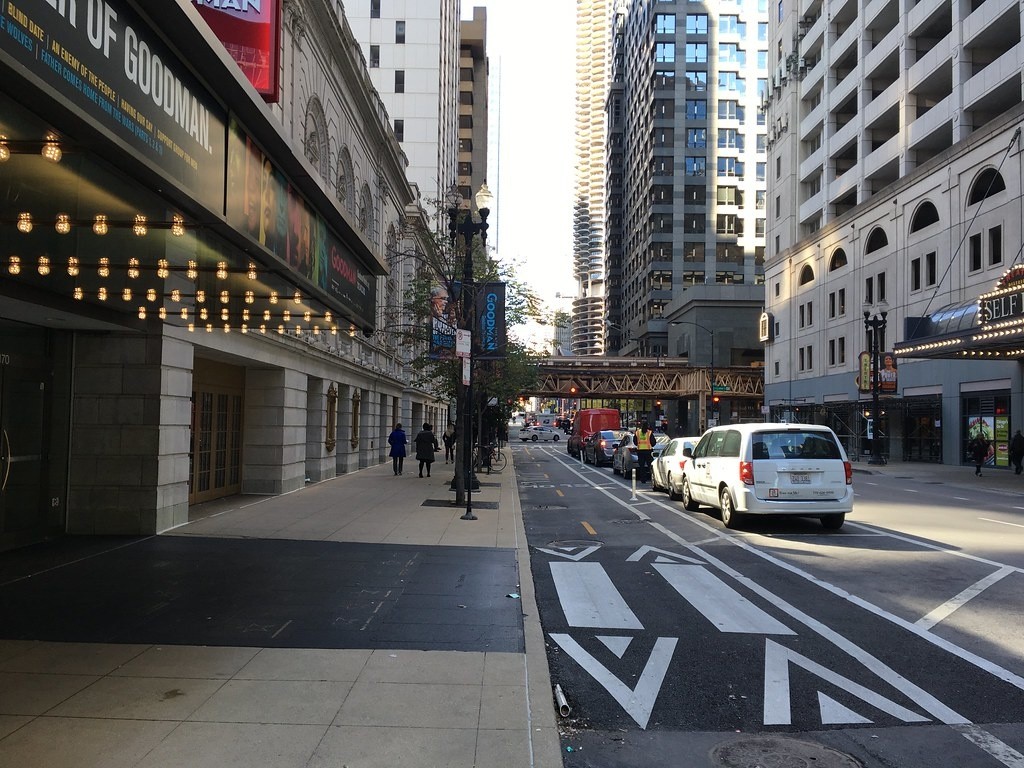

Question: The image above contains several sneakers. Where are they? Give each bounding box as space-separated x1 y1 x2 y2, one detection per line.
978 472 982 477
973 472 978 477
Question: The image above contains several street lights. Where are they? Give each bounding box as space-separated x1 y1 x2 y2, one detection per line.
445 173 496 491
863 295 890 467
671 321 715 419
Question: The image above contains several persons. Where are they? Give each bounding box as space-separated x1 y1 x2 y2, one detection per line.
388 422 407 476
1008 428 1024 475
228 115 329 292
879 354 897 391
634 421 656 483
413 423 436 478
442 423 456 464
969 433 990 478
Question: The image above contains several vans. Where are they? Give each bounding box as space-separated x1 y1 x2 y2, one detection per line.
565 407 620 457
683 423 854 530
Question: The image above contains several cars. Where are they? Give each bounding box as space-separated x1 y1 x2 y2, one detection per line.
585 430 631 468
649 437 702 500
518 426 561 442
612 433 671 479
520 408 575 435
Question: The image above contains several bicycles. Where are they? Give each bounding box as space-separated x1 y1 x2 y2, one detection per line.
472 442 507 472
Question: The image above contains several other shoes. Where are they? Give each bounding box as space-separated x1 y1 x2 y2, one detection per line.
398 473 403 475
427 474 431 477
393 473 398 476
419 474 424 478
446 462 449 464
451 461 454 464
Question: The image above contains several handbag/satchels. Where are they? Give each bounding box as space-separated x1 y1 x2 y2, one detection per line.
451 445 456 450
